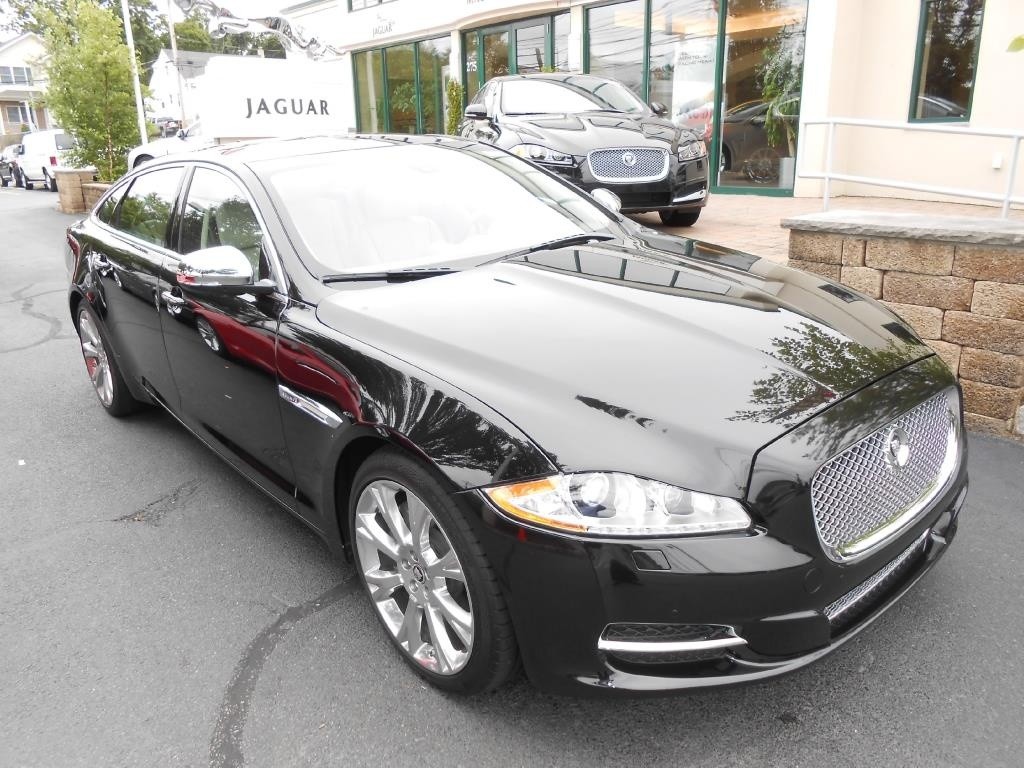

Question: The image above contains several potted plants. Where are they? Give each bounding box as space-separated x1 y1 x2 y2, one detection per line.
754 35 805 189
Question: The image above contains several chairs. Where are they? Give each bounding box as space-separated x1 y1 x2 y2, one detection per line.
200 194 263 281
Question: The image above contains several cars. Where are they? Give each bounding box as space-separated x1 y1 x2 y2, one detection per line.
460 72 710 226
724 91 970 186
66 116 973 696
0 144 25 188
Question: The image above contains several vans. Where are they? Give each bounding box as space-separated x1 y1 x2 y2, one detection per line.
13 129 99 192
